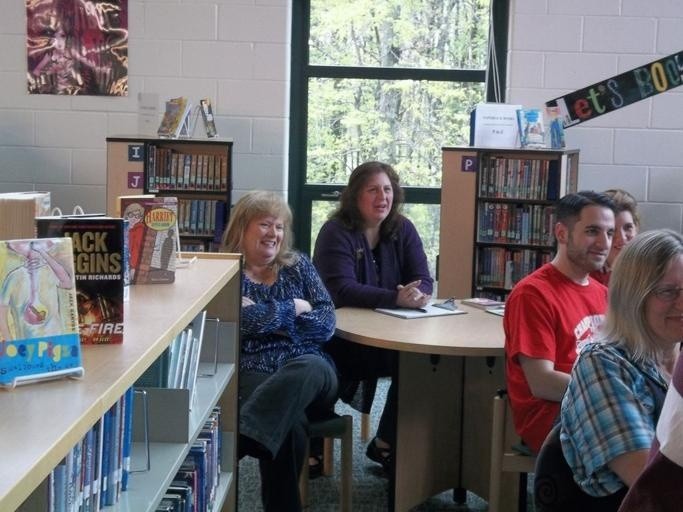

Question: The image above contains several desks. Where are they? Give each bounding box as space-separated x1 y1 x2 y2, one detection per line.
332 299 523 512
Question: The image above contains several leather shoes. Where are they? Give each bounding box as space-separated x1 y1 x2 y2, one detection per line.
309 456 324 480
367 437 396 466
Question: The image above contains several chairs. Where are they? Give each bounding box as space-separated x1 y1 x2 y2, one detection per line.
484 384 546 512
528 419 630 512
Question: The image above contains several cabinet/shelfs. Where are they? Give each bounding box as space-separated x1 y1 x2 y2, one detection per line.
103 133 236 254
435 144 580 306
0 250 243 512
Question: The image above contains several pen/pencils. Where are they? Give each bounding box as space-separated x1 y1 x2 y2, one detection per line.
417 308 426 312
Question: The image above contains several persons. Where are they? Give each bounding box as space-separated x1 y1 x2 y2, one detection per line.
28 15 96 94
503 191 619 460
615 349 682 512
220 192 338 512
311 161 438 481
591 187 641 288
534 228 681 512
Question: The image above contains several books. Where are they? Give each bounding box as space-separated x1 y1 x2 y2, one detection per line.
148 94 231 252
486 304 505 317
117 194 181 284
374 302 469 320
15 310 234 512
0 213 129 385
473 98 566 299
461 296 499 310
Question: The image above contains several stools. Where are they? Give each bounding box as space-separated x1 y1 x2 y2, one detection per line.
345 365 392 444
295 402 360 512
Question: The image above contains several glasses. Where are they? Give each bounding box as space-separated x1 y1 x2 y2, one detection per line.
650 285 683 303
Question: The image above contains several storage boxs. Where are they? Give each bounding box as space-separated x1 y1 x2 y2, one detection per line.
467 101 522 149
